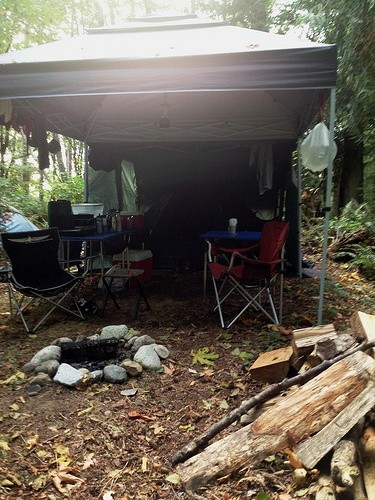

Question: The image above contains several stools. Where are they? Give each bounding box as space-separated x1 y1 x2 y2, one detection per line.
103 268 151 319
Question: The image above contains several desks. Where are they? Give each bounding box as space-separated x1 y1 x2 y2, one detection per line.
200 231 276 304
59 231 129 290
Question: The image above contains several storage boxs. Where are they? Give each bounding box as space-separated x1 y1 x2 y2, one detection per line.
120 211 145 229
113 250 152 287
72 203 104 218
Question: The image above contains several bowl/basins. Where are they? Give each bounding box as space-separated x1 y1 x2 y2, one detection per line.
75 226 96 236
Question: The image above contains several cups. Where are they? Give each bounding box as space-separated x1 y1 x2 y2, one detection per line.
97 215 106 233
228 219 237 234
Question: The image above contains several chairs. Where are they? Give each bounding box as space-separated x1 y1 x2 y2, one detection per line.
0 226 86 333
204 219 291 330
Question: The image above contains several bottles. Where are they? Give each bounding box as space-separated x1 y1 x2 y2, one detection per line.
113 210 121 232
